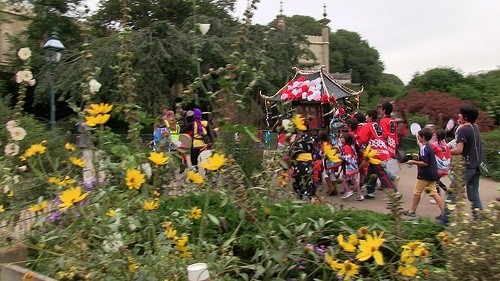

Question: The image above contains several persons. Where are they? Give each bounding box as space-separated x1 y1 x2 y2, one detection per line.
401 127 444 218
179 102 483 225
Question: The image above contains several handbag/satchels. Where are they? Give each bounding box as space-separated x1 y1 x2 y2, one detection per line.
385 158 401 173
479 161 490 176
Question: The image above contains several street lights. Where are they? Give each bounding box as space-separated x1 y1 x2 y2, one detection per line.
42 38 66 130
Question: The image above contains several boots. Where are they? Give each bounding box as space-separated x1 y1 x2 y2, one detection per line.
278 176 288 187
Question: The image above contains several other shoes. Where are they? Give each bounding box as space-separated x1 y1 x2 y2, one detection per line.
402 210 416 217
341 191 353 198
393 176 400 183
436 215 445 220
430 199 437 204
354 194 364 200
363 195 375 199
326 188 338 196
